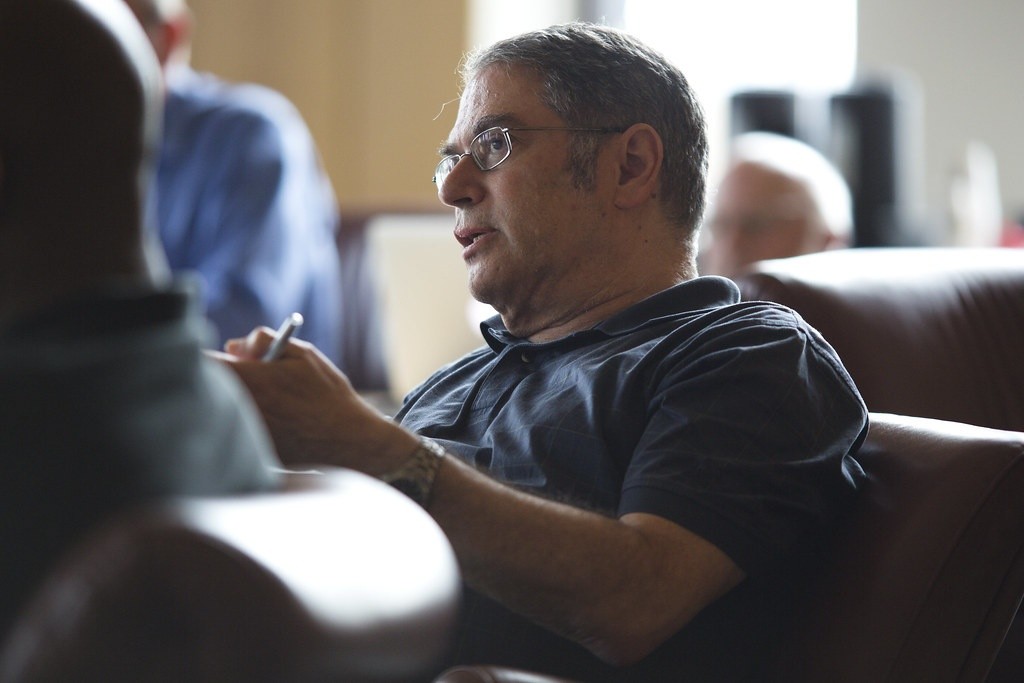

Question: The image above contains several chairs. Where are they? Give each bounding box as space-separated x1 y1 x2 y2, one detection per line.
3 213 1024 682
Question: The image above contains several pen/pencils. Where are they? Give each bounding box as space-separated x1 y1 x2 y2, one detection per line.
261 312 303 363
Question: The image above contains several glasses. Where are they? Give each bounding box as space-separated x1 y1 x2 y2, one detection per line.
433 126 633 196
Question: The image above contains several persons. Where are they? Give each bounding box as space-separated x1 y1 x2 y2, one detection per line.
0 0 277 612
697 130 854 282
130 0 339 361
204 21 870 683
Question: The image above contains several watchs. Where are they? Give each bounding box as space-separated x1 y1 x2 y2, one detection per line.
378 433 444 510
